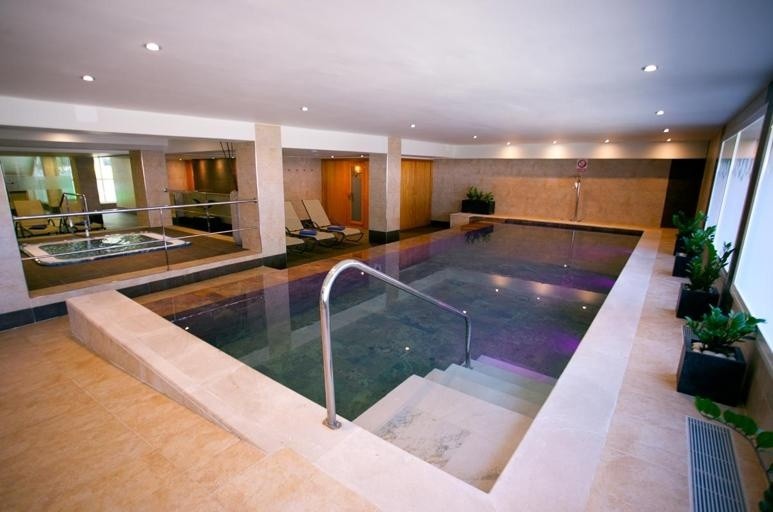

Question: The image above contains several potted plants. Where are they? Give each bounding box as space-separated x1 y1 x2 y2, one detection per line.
677 243 736 322
671 211 718 279
676 308 766 406
460 186 496 215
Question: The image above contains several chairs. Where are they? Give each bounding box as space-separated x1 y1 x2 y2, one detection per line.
11 195 104 238
284 197 365 253
